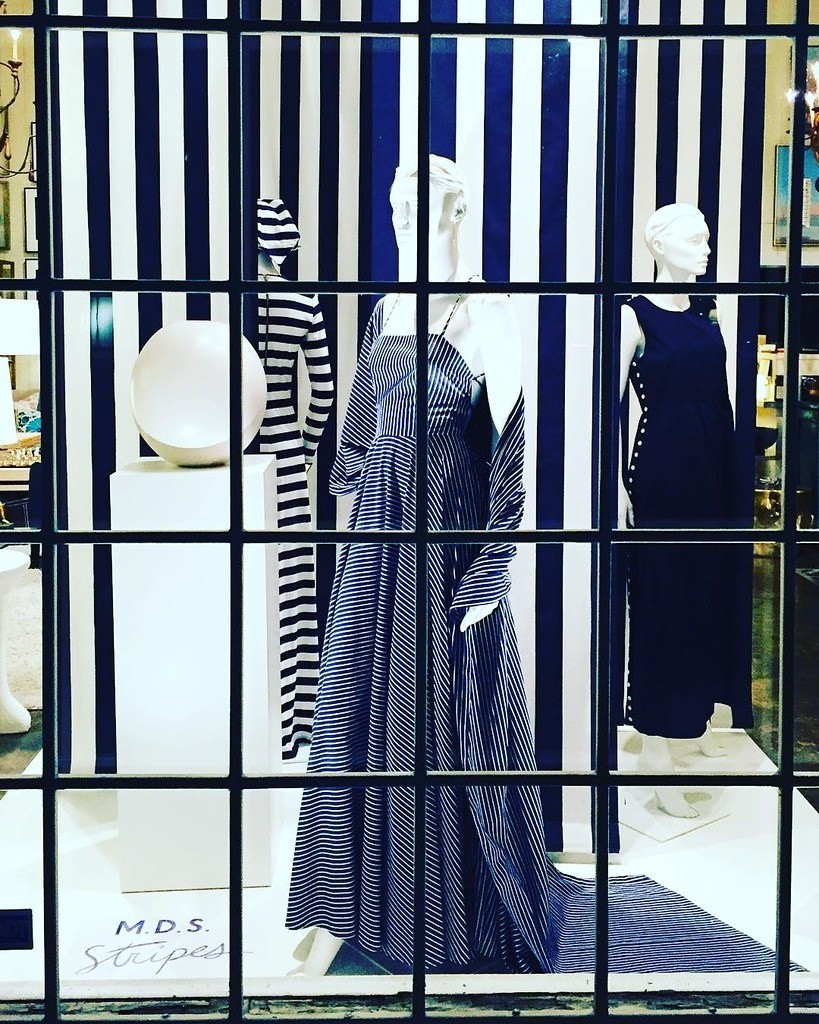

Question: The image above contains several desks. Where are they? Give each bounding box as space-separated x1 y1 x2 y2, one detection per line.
0 550 31 735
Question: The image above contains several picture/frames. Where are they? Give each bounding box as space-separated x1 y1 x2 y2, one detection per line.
24 187 40 253
30 121 37 183
790 45 819 84
772 143 819 251
24 258 39 299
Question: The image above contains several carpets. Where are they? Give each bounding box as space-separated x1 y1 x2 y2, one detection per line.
3 546 44 709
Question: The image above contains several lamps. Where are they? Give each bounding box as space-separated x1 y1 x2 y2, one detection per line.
786 106 819 195
0 30 37 180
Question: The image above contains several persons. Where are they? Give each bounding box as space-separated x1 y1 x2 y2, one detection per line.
9 361 39 429
614 201 758 818
253 197 333 761
284 152 809 999
